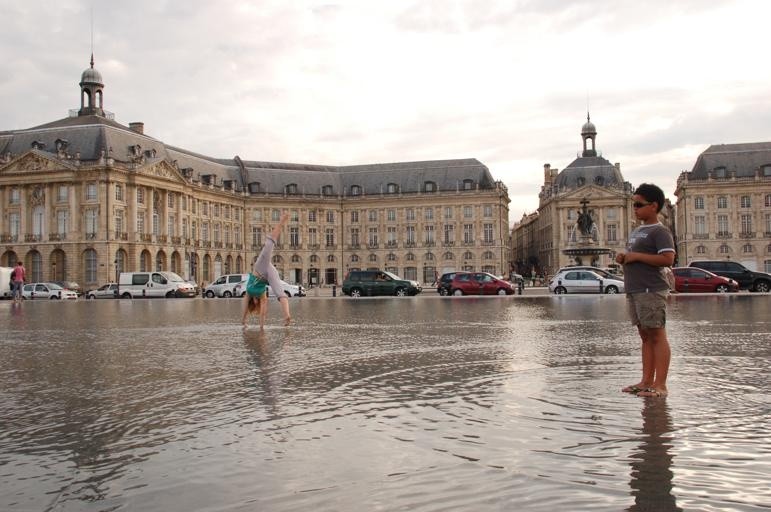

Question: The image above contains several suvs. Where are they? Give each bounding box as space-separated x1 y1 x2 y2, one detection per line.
555 265 624 281
688 259 771 293
342 270 422 297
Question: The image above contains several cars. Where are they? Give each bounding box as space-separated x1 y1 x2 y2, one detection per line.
21 282 78 300
669 267 741 293
88 282 119 298
436 271 468 296
548 269 626 294
452 272 516 296
46 280 82 295
236 275 307 297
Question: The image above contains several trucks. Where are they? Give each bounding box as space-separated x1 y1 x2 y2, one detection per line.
1 266 25 300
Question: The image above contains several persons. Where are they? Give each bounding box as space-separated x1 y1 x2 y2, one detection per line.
241 211 291 330
9 266 20 300
12 262 26 304
615 184 676 397
531 270 548 287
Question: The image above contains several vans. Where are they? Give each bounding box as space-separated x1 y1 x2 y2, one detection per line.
118 270 196 297
203 274 250 297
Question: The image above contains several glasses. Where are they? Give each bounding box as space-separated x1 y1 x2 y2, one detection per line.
634 201 651 208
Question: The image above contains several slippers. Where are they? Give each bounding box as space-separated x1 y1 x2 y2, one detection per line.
622 385 669 398
